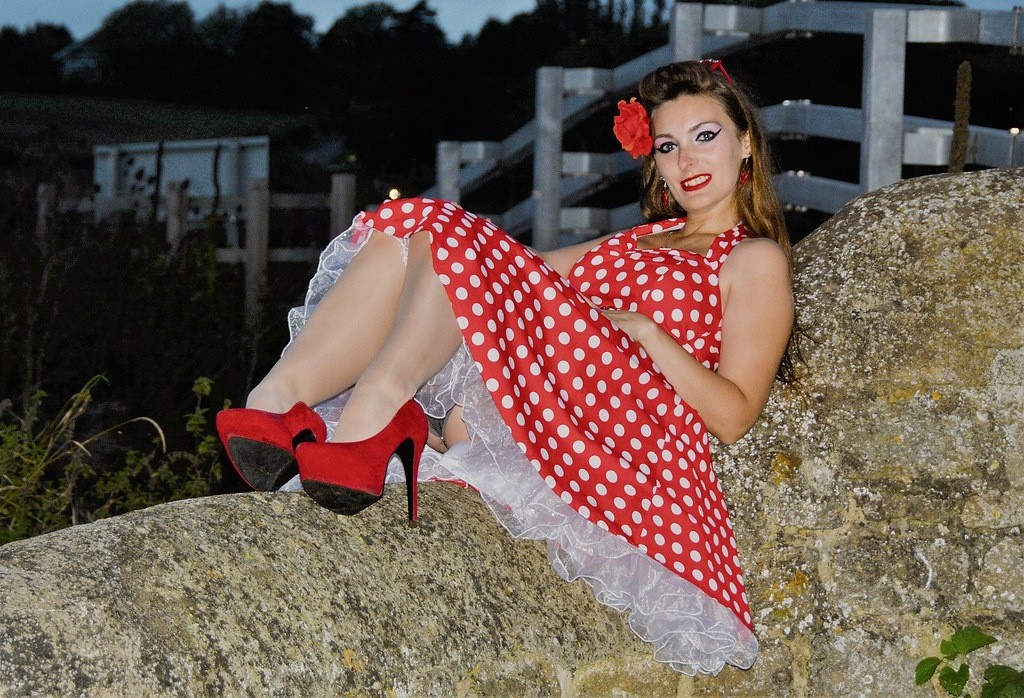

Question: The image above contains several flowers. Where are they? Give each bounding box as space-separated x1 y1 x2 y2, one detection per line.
612 97 654 159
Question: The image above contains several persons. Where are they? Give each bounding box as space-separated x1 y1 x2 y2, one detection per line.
217 61 797 678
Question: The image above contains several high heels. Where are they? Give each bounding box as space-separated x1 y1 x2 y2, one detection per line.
295 400 429 528
216 401 327 492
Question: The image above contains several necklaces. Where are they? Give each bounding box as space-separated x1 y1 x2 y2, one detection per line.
666 229 711 249
